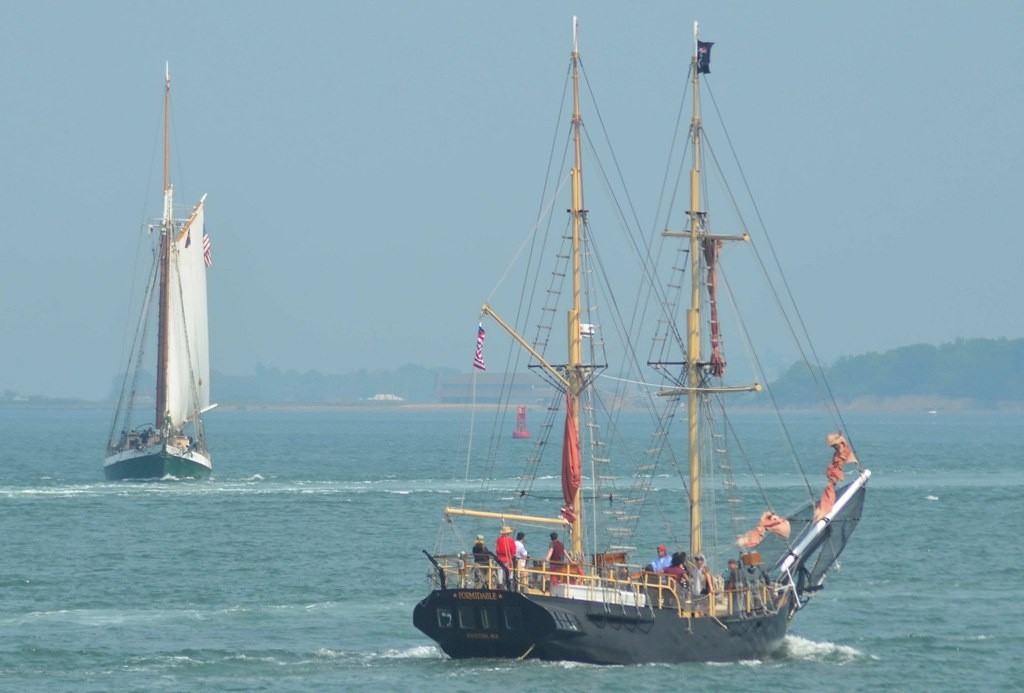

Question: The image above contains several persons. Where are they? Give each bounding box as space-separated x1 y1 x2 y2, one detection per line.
189 438 199 452
644 545 770 600
496 527 516 592
472 534 494 581
114 427 156 452
514 532 528 590
545 533 577 593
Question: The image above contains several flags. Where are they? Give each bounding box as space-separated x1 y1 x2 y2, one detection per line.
203 223 212 268
698 39 715 73
474 322 486 370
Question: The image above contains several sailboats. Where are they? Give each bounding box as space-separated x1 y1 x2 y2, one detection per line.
414 13 872 667
102 57 217 483
514 406 530 437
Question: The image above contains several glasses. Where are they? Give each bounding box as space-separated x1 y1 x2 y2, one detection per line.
658 550 661 552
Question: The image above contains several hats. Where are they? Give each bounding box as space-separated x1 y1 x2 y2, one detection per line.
672 552 685 564
728 560 734 564
695 554 703 560
500 526 512 534
516 532 525 539
549 533 557 538
657 545 665 551
474 534 484 544
